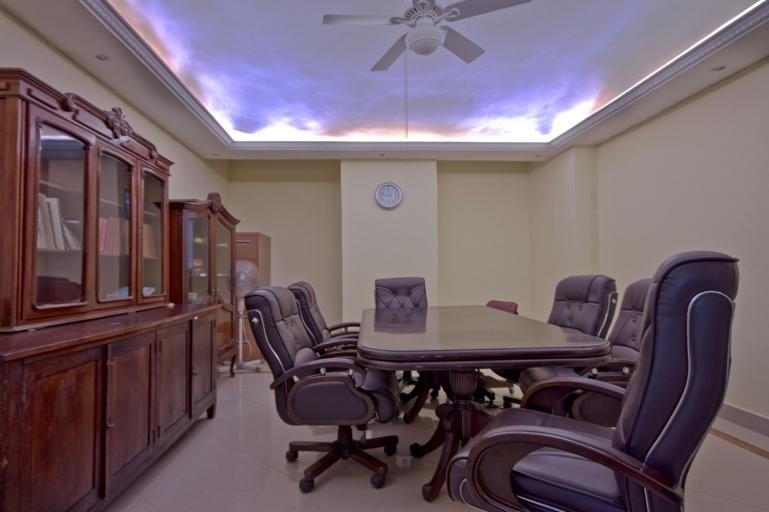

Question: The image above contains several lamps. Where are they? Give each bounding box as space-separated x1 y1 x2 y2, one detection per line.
404 17 445 57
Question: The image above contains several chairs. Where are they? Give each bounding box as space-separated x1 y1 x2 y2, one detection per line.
485 299 519 392
288 281 395 430
374 276 440 397
519 279 655 428
490 274 618 409
444 249 740 512
244 285 399 494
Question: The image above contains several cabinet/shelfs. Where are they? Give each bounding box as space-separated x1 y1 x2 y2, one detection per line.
168 189 240 380
235 229 270 364
0 68 177 334
1 301 225 512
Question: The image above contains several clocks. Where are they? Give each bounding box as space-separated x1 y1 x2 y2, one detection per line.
375 181 402 210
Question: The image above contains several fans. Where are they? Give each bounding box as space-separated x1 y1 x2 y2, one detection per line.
322 0 531 72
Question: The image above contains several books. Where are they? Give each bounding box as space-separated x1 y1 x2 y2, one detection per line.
32 183 160 262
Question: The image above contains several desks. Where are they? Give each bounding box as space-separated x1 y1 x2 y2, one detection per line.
357 305 613 502
